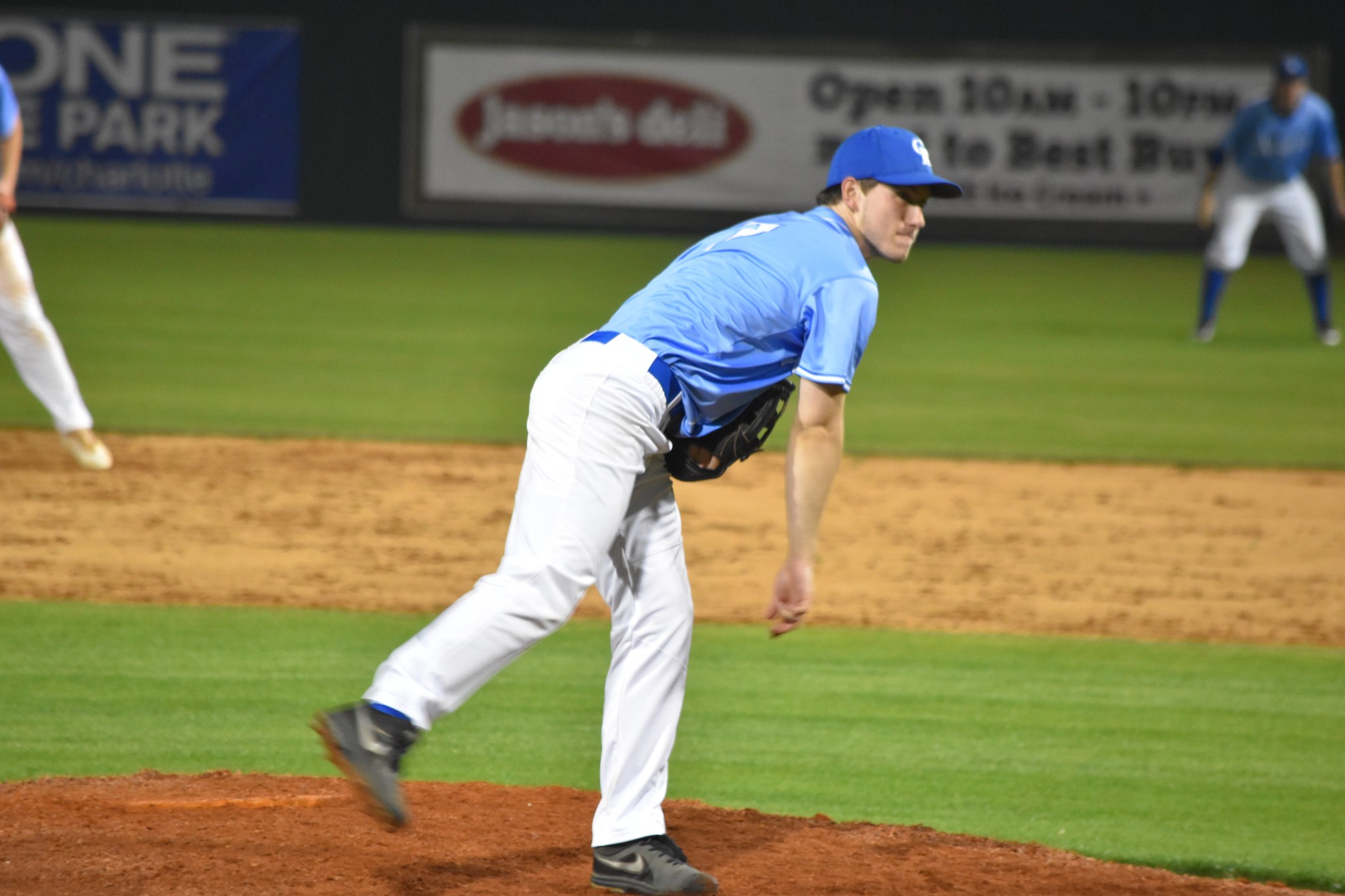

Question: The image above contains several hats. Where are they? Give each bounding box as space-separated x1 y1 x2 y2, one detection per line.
1278 58 1306 82
826 124 964 198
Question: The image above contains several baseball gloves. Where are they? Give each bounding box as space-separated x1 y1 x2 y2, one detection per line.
664 375 796 481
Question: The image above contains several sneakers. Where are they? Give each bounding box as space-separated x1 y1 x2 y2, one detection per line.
590 834 719 896
313 698 412 830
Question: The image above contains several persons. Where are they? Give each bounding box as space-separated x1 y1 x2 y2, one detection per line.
311 124 962 896
1193 55 1344 344
0 68 111 470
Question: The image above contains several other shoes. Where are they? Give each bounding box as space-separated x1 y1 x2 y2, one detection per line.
1318 326 1340 345
66 427 114 471
1194 323 1214 342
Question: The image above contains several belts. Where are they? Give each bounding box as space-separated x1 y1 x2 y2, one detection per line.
584 329 685 439
